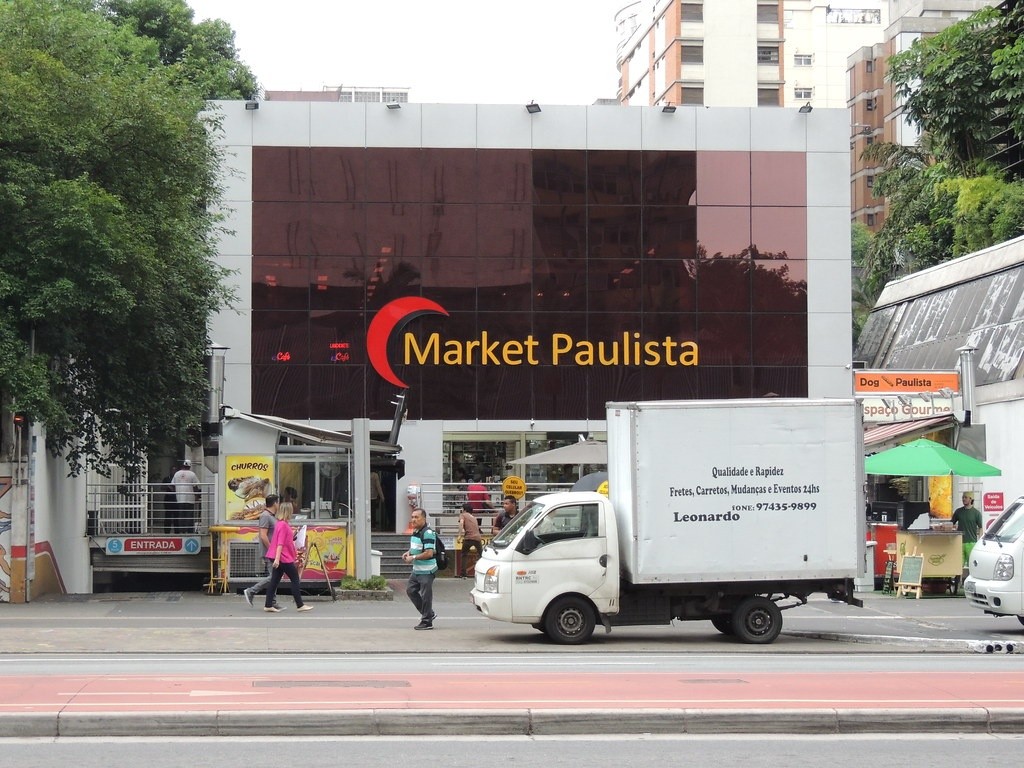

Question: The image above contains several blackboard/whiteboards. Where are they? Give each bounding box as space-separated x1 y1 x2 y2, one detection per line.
898 555 924 587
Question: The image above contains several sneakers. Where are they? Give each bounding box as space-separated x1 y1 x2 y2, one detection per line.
432 613 437 620
460 575 467 579
274 605 287 611
244 589 253 607
297 605 314 613
414 621 434 630
264 607 279 612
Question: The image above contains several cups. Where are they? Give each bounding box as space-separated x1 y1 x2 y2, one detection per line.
882 511 887 523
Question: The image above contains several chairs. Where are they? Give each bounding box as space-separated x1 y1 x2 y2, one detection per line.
582 512 596 537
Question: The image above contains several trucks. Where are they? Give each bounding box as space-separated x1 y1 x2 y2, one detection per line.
470 397 867 645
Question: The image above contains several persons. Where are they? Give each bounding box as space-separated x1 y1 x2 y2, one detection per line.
282 487 301 513
172 460 200 533
244 494 281 612
263 502 314 612
161 466 180 534
467 474 497 514
946 490 983 584
454 467 469 491
473 456 493 476
402 508 438 630
457 504 484 580
369 466 385 531
492 495 522 534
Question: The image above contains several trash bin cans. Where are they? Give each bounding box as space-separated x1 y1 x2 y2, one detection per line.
852 540 878 593
371 549 383 576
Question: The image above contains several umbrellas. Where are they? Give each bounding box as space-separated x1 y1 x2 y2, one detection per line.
864 436 1001 501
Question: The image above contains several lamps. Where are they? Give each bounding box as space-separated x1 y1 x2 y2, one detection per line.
897 395 912 418
244 96 259 110
846 363 851 370
387 100 400 109
662 101 677 112
938 387 954 412
919 392 935 414
526 99 541 113
881 398 896 420
798 100 812 113
531 419 534 427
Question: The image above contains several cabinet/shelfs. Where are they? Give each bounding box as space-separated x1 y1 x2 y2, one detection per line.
441 442 517 508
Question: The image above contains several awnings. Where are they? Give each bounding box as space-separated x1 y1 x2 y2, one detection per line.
864 412 958 445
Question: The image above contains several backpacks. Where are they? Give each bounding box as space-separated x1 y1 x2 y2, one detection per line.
420 528 447 569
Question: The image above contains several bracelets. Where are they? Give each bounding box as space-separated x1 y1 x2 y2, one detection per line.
413 555 417 560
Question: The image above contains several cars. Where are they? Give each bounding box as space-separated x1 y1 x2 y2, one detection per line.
963 497 1024 626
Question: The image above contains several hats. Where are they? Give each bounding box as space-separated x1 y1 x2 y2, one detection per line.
963 491 974 499
183 460 192 466
